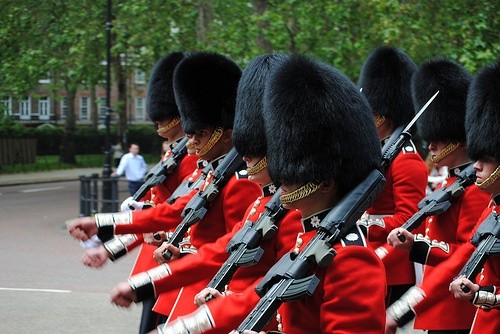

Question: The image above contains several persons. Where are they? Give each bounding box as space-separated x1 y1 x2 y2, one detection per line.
110 144 147 197
65 48 500 334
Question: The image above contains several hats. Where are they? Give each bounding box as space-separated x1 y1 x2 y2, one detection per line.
359 45 419 126
465 59 499 158
147 50 179 121
261 51 383 191
232 51 281 159
410 56 475 144
173 50 243 135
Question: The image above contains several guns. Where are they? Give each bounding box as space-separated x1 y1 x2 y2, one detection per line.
127 136 190 210
452 209 500 293
161 146 246 260
234 90 441 333
380 117 418 173
394 159 477 243
202 184 286 302
